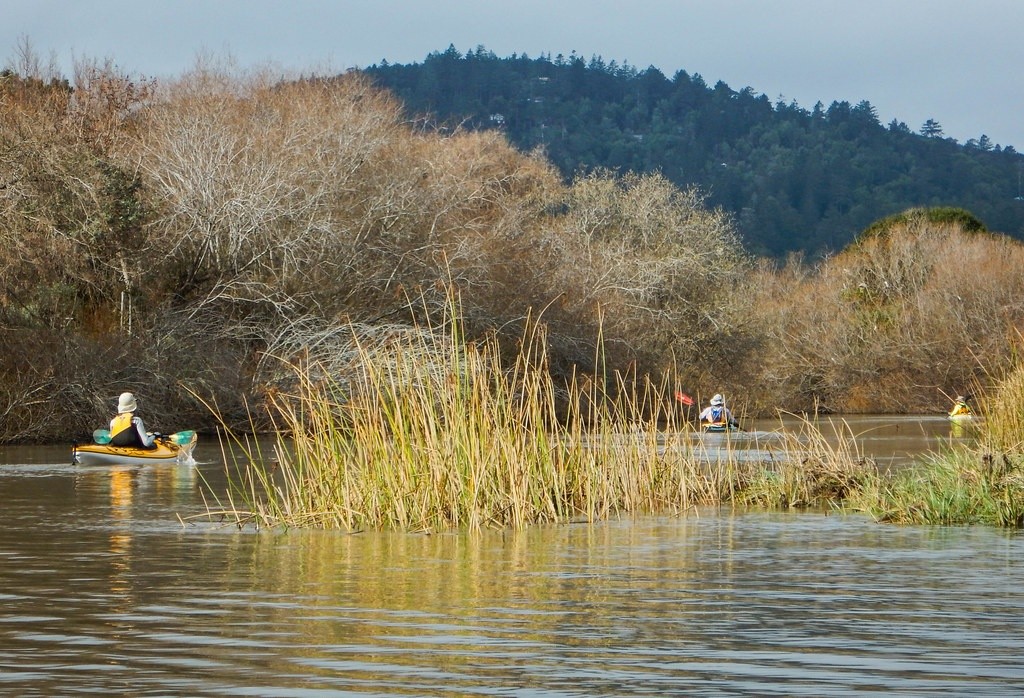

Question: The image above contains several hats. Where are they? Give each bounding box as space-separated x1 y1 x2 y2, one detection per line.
118 392 138 414
955 395 965 402
710 393 724 406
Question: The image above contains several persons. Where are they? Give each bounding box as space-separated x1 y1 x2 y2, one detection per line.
951 419 965 437
109 392 163 450
951 395 972 416
698 393 742 429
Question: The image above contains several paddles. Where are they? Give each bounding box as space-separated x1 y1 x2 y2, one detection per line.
92 428 197 446
674 390 748 433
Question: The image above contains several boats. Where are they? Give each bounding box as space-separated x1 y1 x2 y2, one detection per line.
703 421 732 432
71 430 199 467
948 412 972 422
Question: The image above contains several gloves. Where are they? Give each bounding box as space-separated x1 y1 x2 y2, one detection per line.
153 431 163 439
